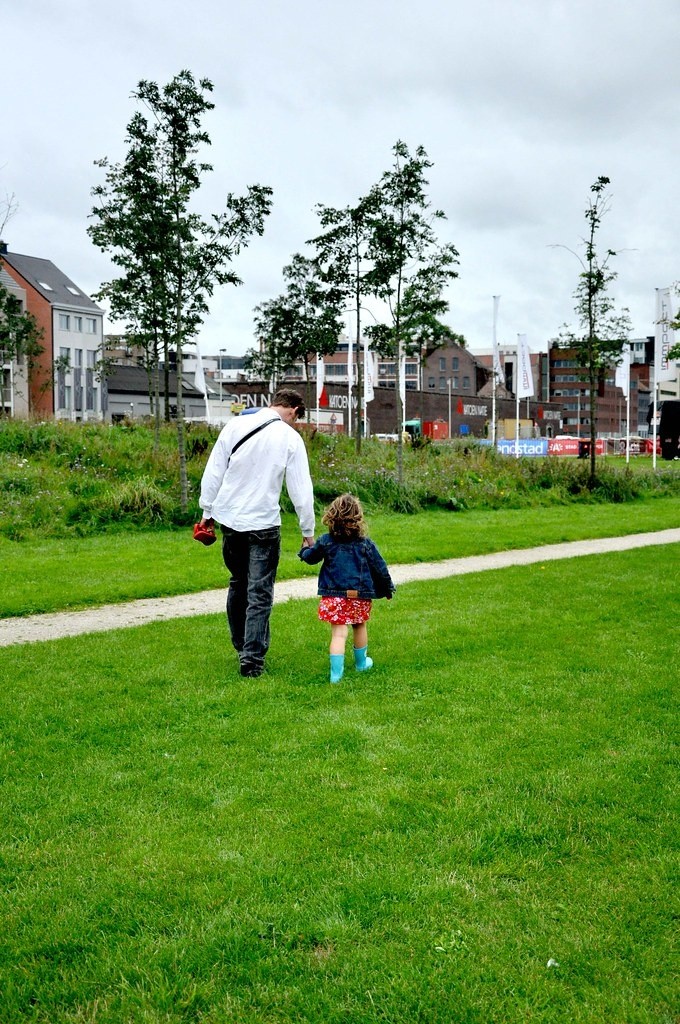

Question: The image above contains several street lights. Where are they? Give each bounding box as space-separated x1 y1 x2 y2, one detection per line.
219 349 226 416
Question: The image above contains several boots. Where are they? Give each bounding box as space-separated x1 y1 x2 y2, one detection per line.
330 653 344 683
353 644 373 670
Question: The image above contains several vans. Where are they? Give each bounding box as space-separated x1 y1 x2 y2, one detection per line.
398 419 421 440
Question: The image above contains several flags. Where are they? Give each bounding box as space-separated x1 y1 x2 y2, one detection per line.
654 288 674 383
194 351 207 395
398 342 406 404
364 340 374 402
516 341 535 399
316 354 328 409
492 341 505 390
614 342 628 399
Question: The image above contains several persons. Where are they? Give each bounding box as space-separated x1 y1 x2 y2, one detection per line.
196 387 315 678
296 494 395 686
